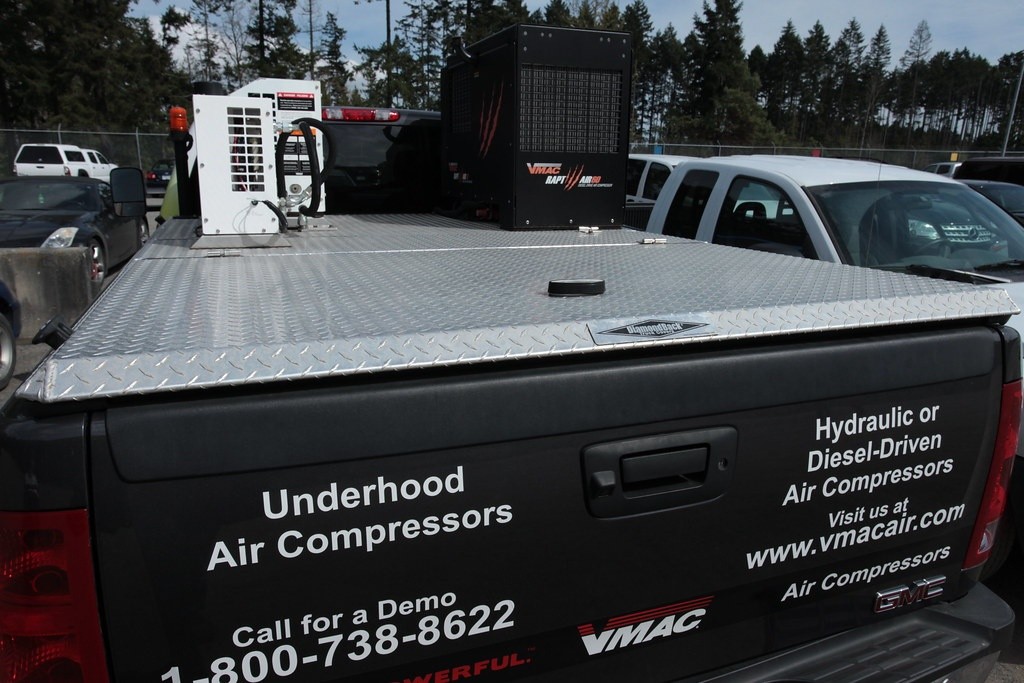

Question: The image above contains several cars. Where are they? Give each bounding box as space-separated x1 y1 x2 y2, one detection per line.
145 160 175 195
0 175 151 288
954 179 1024 229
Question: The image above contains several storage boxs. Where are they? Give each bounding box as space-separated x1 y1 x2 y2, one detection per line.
441 22 631 232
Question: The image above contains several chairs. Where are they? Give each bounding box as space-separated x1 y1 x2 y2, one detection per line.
732 199 767 227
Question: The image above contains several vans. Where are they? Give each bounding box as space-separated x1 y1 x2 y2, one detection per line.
13 143 119 185
626 154 704 203
922 157 1024 187
645 154 1024 458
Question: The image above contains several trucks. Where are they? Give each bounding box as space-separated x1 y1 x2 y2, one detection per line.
2 106 1023 683
